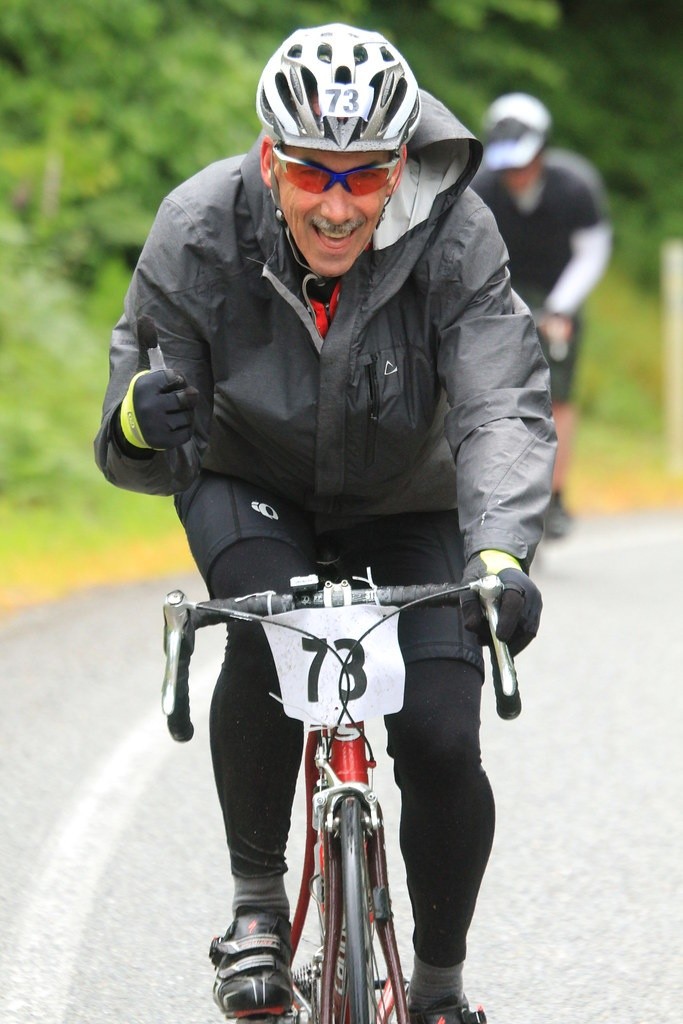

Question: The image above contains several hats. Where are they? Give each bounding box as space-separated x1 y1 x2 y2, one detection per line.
483 133 545 173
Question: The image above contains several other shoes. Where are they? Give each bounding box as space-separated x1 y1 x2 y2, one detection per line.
539 491 568 541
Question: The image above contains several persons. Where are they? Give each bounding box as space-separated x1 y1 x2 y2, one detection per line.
470 93 613 539
92 22 562 1024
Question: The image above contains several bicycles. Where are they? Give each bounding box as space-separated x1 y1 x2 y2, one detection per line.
159 572 525 1023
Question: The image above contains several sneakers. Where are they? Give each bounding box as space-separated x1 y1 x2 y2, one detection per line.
209 905 294 1018
404 985 487 1024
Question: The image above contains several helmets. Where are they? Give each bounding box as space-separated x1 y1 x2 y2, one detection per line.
256 24 420 152
478 93 552 139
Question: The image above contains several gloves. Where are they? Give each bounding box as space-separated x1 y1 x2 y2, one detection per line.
459 547 542 657
109 313 199 460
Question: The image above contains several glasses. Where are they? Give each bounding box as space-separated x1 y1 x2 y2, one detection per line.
273 140 403 195
